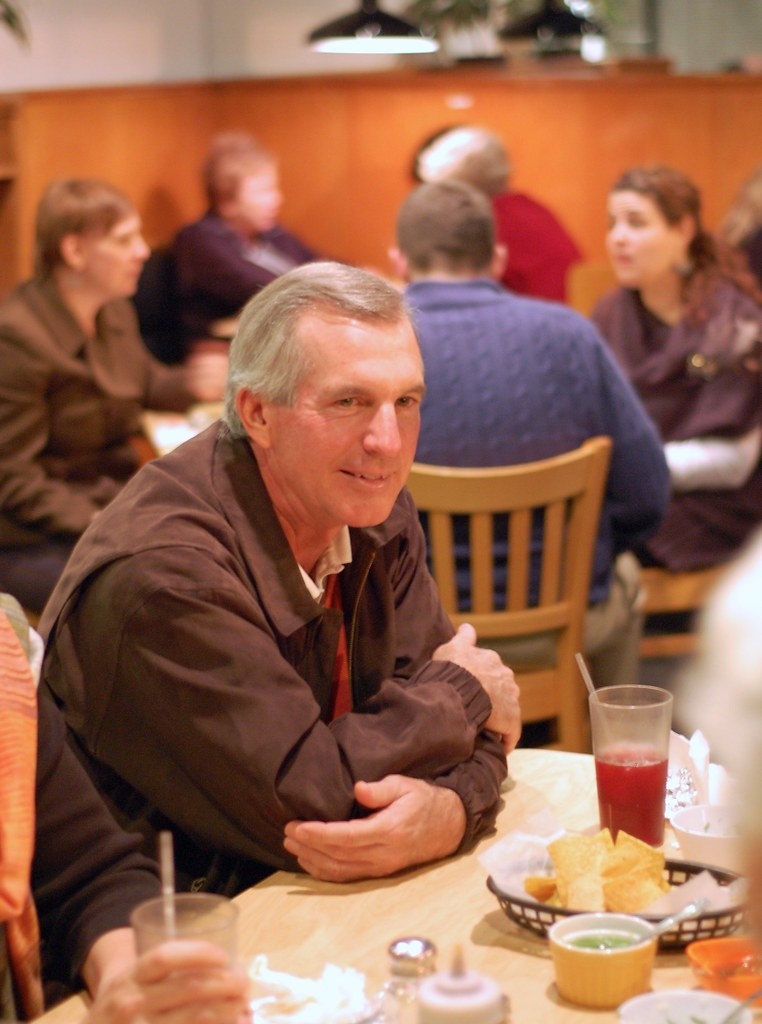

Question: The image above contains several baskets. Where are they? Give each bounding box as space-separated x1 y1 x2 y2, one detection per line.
487 858 747 952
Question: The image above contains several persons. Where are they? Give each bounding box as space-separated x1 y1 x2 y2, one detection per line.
0 178 229 617
36 260 521 899
0 593 251 1024
589 163 762 634
389 182 670 695
137 134 322 370
388 122 584 303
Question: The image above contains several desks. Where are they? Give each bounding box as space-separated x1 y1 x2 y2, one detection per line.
16 744 709 1024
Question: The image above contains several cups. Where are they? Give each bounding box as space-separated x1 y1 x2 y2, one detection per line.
589 684 675 848
618 989 753 1024
130 892 239 970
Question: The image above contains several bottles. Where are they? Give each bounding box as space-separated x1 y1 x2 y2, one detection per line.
382 936 438 1023
417 944 503 1023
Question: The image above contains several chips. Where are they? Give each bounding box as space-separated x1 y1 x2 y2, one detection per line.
521 827 674 913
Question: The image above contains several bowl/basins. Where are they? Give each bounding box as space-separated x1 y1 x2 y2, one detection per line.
670 803 743 875
547 913 658 1010
687 935 762 1008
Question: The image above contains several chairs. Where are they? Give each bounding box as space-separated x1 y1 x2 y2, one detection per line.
413 436 611 749
0 587 50 1024
640 565 732 679
